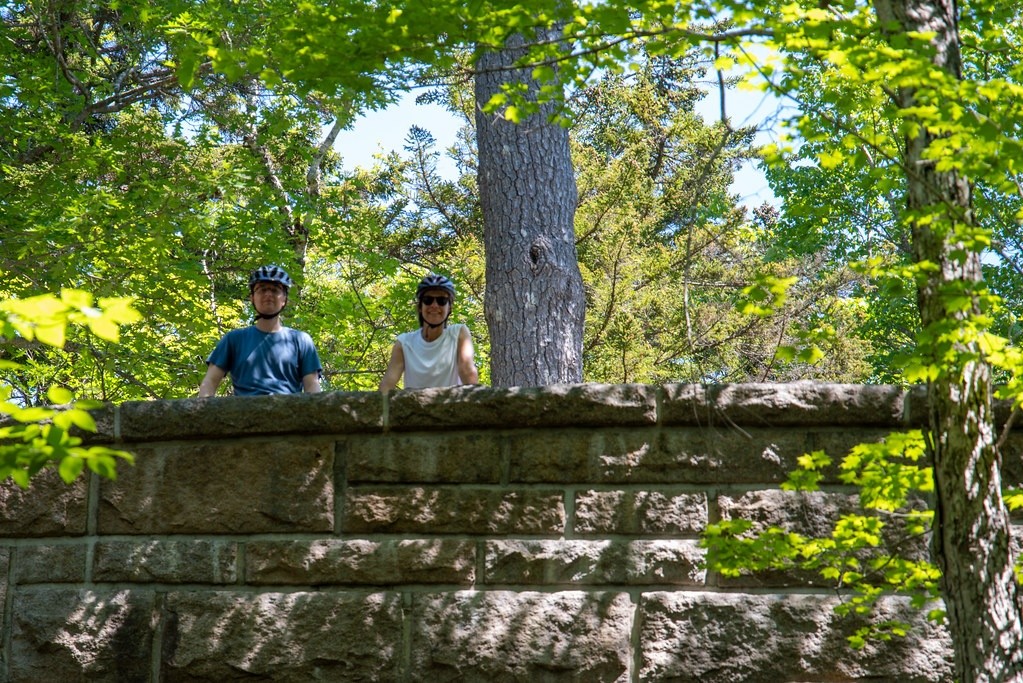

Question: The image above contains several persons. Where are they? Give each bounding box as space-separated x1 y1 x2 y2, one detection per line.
200 265 323 397
379 275 478 388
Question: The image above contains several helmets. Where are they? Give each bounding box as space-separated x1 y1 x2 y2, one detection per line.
417 276 455 296
249 264 296 287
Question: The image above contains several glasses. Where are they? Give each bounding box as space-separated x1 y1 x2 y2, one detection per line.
254 285 286 295
421 295 450 306
